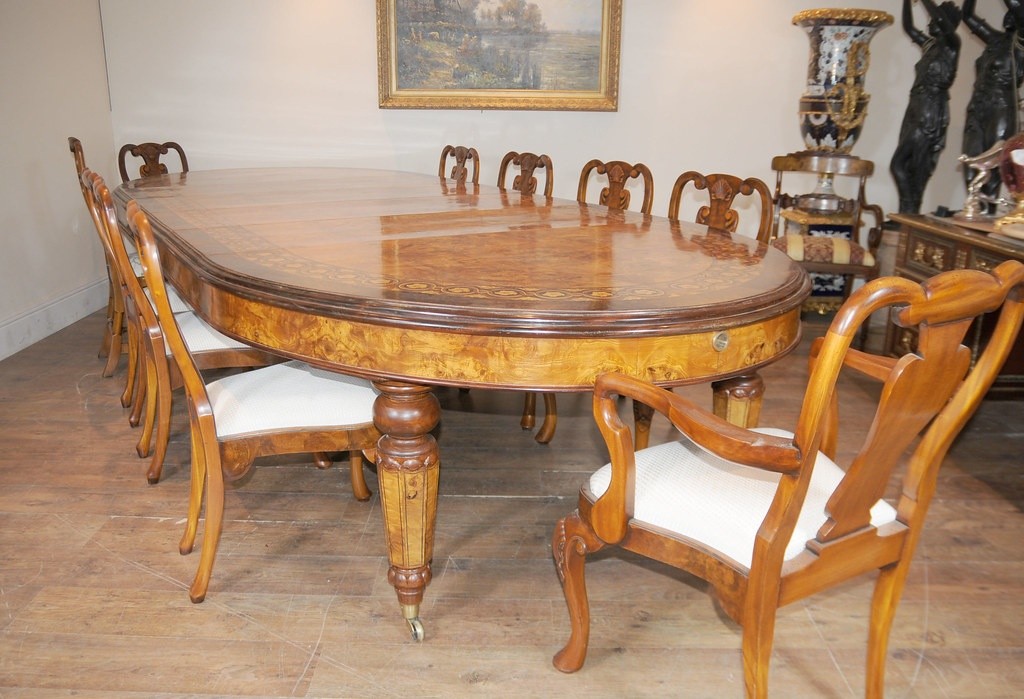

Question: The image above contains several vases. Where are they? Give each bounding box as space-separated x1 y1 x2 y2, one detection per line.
782 9 890 313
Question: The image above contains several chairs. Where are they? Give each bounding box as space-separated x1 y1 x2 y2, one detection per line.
434 144 1024 699
68 137 382 603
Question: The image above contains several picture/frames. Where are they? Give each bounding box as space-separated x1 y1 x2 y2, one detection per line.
375 0 622 112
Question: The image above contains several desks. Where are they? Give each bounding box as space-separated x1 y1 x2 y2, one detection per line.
880 212 1024 454
108 165 817 644
